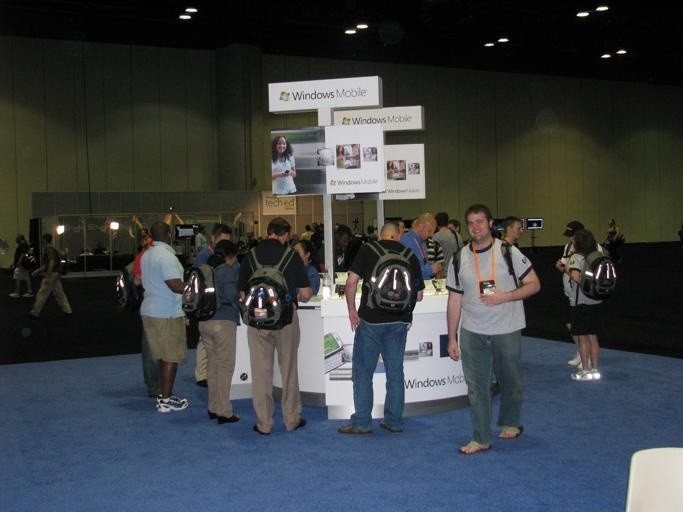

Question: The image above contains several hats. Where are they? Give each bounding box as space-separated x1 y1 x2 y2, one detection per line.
563 220 584 237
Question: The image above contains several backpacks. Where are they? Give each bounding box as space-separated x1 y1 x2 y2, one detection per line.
365 239 417 314
182 263 236 321
579 251 616 301
117 266 139 312
242 246 296 328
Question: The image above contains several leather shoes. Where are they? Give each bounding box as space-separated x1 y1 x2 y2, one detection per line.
294 419 306 429
253 423 270 434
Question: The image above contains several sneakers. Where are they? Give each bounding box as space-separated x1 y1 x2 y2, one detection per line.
22 292 34 297
577 360 592 369
337 424 373 435
379 419 403 433
570 371 593 381
159 400 187 412
156 394 188 407
217 416 240 423
567 354 581 366
9 293 21 297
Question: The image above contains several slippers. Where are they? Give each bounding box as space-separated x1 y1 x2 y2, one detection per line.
498 426 523 439
460 441 491 453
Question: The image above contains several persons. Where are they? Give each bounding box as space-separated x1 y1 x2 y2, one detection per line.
371 147 377 160
138 222 192 414
568 227 605 383
194 212 523 297
133 229 160 399
200 239 242 425
336 217 426 436
235 216 314 435
388 161 396 179
337 145 347 168
8 233 73 320
602 216 623 265
188 224 233 388
347 145 360 168
396 160 406 179
270 135 302 196
444 204 543 456
553 220 593 369
